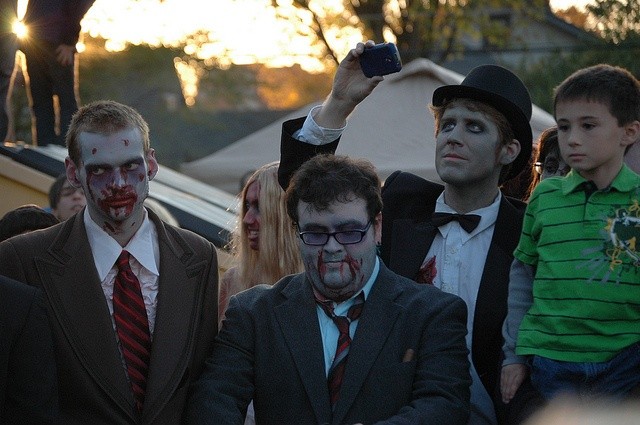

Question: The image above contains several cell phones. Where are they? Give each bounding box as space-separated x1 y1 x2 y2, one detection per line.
359 42 402 78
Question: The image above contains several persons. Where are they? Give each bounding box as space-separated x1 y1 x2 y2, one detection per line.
0 100 220 424
0 206 59 240
527 127 569 192
501 64 640 403
277 40 526 425
185 153 472 423
215 161 306 327
49 172 87 221
1 0 18 143
20 0 94 146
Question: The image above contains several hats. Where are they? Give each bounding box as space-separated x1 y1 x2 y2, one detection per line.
432 65 532 181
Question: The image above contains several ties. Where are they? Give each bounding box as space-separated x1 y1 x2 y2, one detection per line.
313 296 365 421
112 250 152 420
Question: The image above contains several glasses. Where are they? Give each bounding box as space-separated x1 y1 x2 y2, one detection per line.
534 158 570 175
295 215 376 246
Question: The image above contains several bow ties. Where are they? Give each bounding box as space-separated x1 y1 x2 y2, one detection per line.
430 212 481 234
